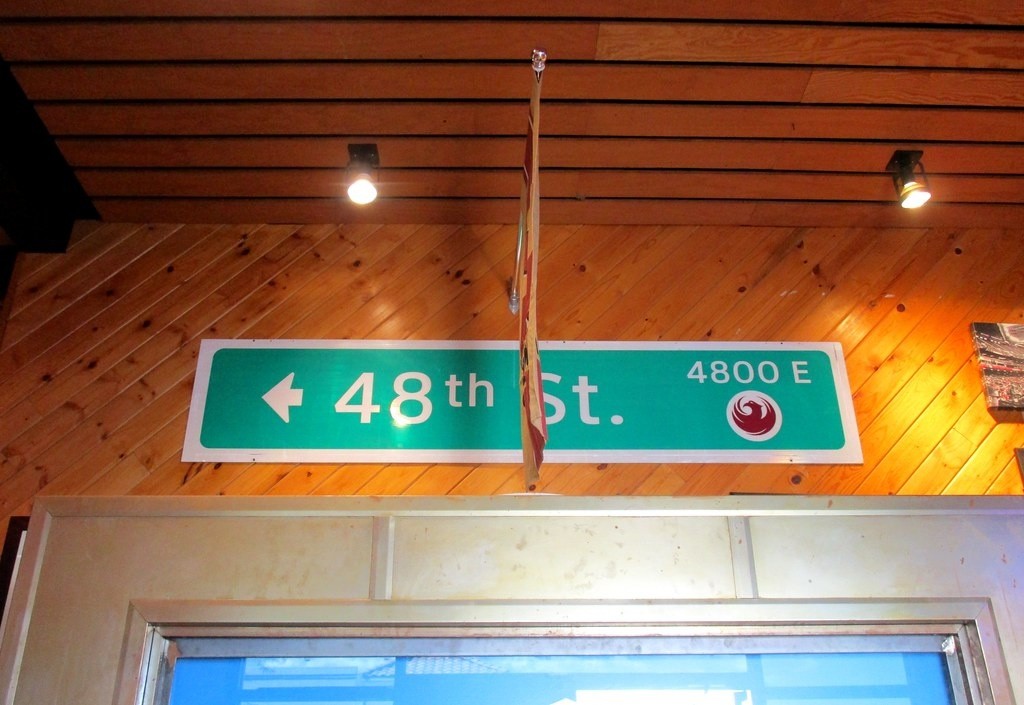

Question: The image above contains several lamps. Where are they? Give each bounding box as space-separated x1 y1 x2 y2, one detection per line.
886 149 933 209
343 144 379 205
